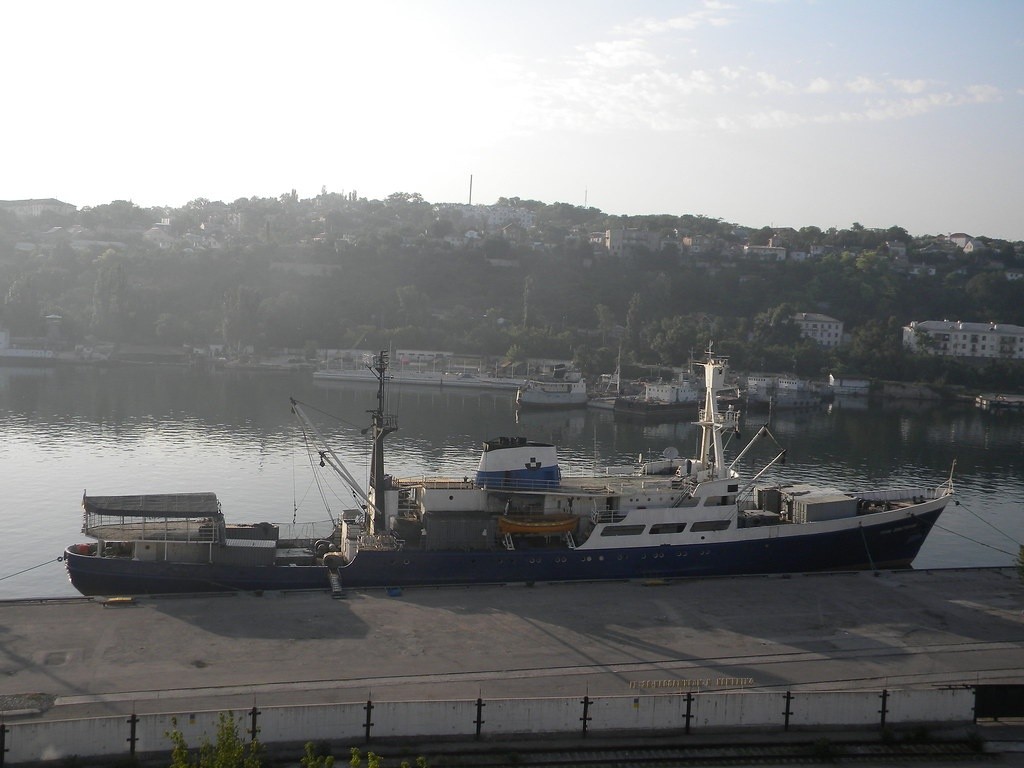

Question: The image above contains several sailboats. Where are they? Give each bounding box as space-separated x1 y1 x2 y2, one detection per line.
588 345 622 411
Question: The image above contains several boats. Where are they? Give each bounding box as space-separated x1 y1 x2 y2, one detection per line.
516 377 588 408
61 339 959 596
